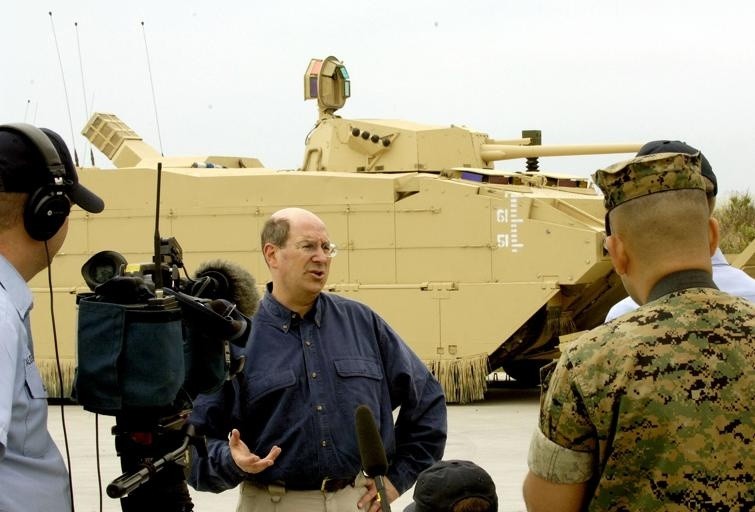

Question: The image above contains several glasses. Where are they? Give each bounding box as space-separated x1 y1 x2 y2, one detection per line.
280 240 338 257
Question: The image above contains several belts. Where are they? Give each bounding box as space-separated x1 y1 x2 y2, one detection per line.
246 477 353 492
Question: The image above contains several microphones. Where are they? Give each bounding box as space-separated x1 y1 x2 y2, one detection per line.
353 405 388 478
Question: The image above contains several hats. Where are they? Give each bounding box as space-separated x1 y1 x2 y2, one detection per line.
1 126 104 213
635 140 718 198
591 151 706 236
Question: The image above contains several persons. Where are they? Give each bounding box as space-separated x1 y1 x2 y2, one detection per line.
599 138 755 325
522 151 755 512
0 123 105 512
185 207 449 510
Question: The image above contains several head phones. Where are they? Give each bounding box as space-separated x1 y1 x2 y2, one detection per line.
0 124 74 243
